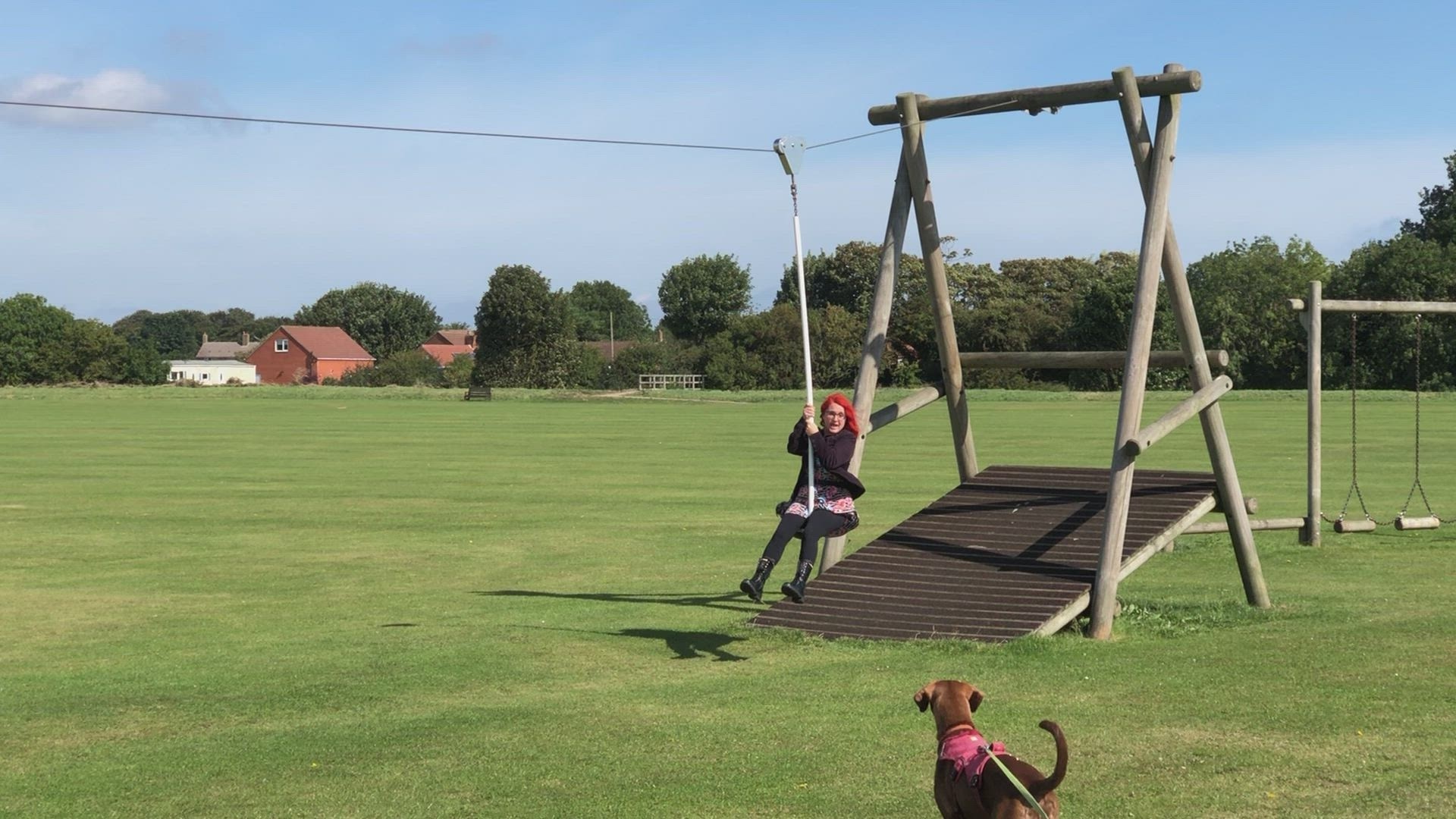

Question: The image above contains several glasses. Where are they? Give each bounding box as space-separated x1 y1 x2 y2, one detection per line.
823 410 846 420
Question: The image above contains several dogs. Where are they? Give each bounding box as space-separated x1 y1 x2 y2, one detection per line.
914 680 1069 819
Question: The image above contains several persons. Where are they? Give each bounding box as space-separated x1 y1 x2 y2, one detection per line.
740 392 866 604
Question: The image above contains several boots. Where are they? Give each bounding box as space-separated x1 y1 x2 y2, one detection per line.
781 559 812 603
740 557 773 601
